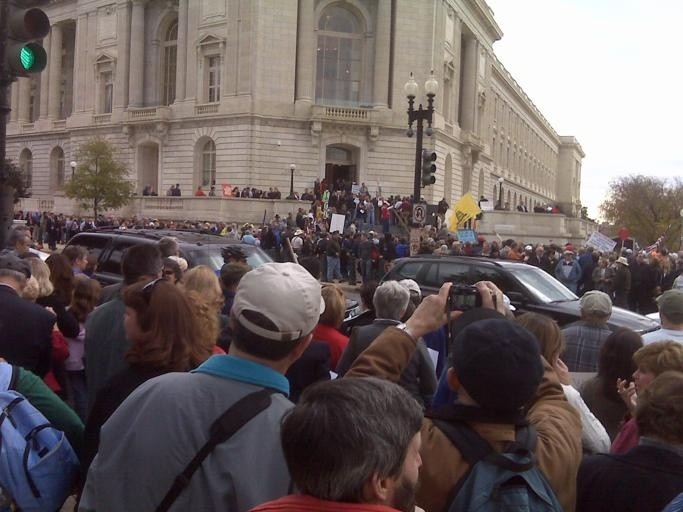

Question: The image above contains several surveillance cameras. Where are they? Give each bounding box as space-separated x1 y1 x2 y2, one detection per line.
277 140 281 147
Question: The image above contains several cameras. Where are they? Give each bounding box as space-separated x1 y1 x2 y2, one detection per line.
447 285 482 311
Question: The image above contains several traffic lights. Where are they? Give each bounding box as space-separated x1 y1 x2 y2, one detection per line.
0 6 51 78
421 151 436 186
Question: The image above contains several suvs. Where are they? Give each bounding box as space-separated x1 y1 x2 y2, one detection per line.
371 254 662 334
61 227 364 328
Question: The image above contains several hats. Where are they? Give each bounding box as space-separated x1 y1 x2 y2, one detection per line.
580 290 612 317
1 255 31 277
294 229 303 236
616 257 629 266
453 306 543 408
399 278 421 297
230 262 325 342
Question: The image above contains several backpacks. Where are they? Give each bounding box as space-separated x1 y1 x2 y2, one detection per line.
0 360 84 512
427 411 563 511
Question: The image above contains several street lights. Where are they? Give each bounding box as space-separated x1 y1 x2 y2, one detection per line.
403 68 438 255
285 164 296 199
70 161 77 177
497 177 504 208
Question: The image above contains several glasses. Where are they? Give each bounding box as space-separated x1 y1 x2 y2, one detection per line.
139 277 171 315
